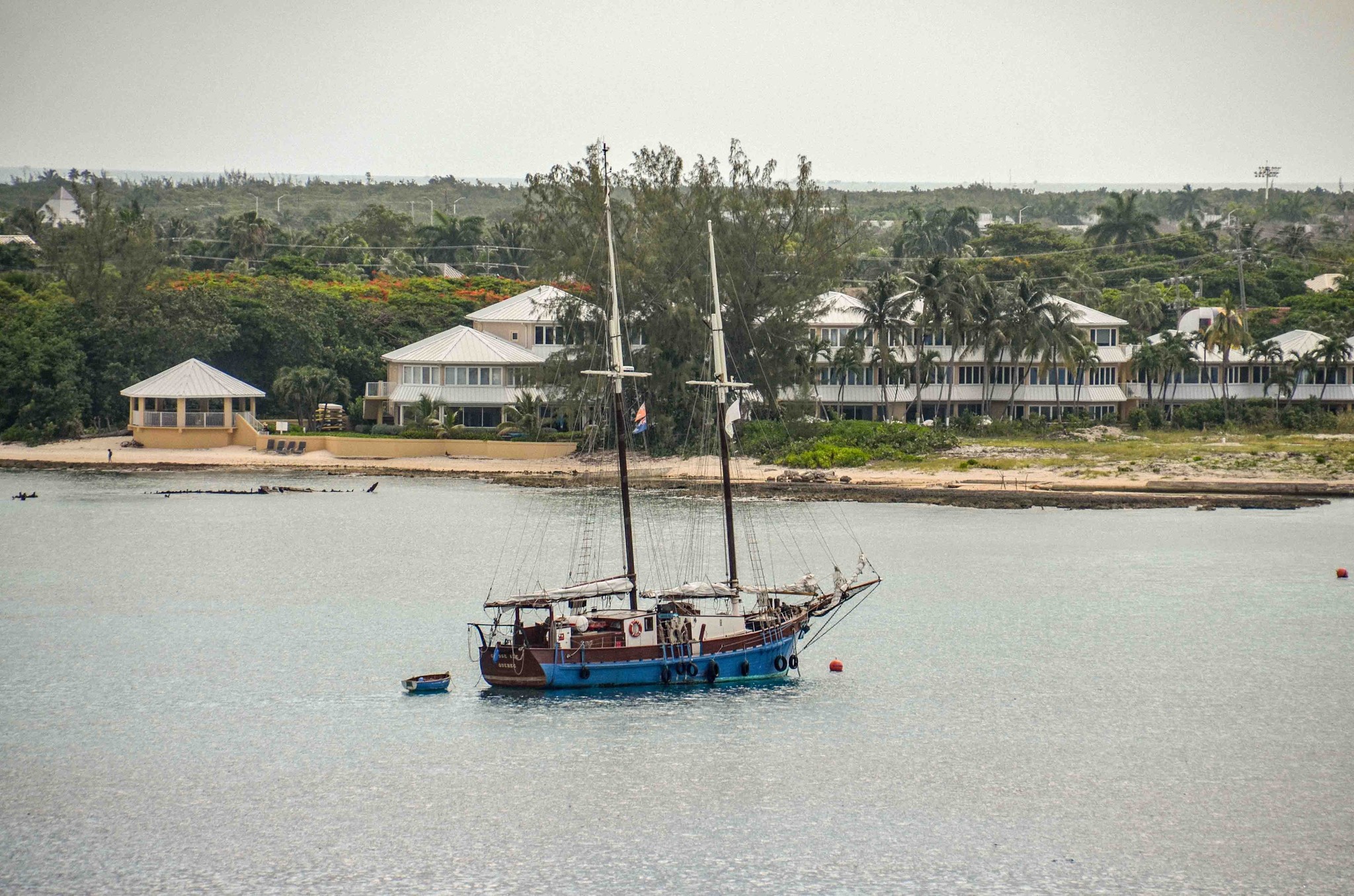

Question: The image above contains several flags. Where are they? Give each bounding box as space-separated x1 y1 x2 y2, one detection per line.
634 401 646 422
725 399 740 438
632 416 647 434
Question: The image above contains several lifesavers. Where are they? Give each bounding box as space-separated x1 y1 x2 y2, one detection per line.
661 669 672 683
675 662 686 674
710 661 719 677
579 668 590 679
742 660 749 676
789 655 798 669
774 655 787 672
629 621 642 637
686 663 698 676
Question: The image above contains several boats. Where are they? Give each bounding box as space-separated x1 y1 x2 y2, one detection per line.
401 674 451 691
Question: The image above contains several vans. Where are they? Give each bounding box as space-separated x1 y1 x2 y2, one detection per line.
552 420 569 432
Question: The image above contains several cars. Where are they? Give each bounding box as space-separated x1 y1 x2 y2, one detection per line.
502 433 522 440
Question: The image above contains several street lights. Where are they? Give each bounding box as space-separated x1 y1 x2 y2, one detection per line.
1228 208 1242 227
1020 206 1031 225
1254 160 1282 213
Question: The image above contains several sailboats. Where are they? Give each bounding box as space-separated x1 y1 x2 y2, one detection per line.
467 143 882 689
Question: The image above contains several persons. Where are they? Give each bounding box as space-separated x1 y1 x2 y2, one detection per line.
28 492 35 497
108 449 112 464
19 492 22 497
21 492 26 500
